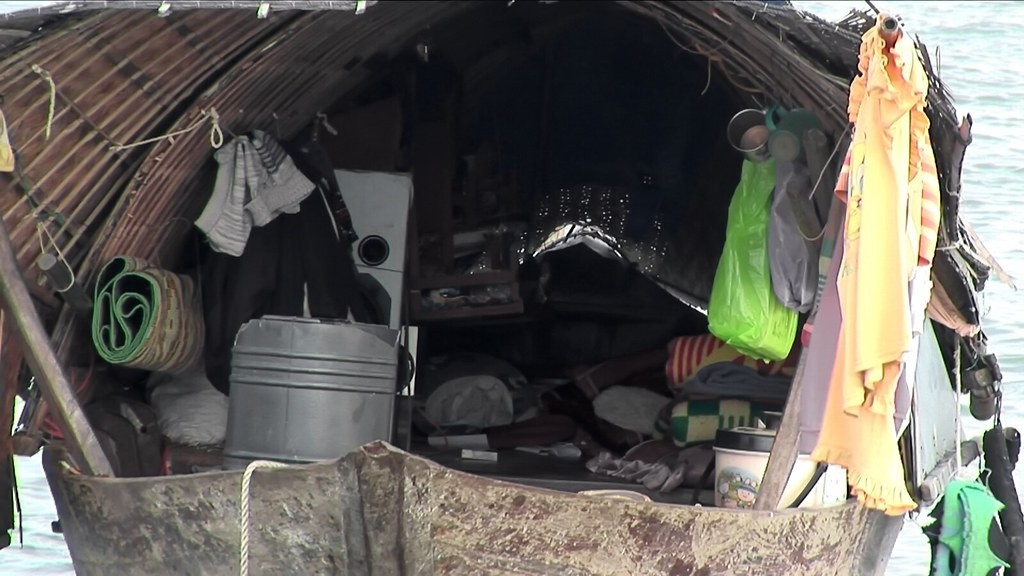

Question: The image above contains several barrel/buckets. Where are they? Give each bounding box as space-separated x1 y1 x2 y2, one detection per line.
220 312 400 474
713 427 848 512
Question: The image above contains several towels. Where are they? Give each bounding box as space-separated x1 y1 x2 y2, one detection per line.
591 386 673 434
192 128 316 257
673 362 792 403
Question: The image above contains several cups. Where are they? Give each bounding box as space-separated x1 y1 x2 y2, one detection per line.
764 103 823 163
726 106 771 163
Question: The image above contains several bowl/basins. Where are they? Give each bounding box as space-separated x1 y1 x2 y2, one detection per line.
593 408 653 455
